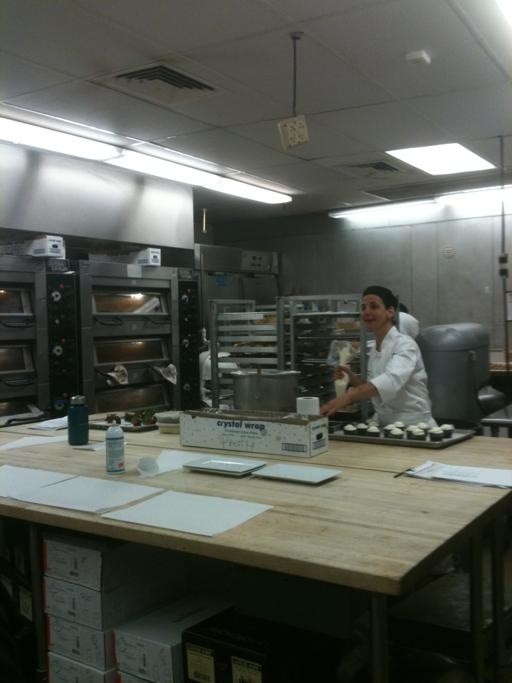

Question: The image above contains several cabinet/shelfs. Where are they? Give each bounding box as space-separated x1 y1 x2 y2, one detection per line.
209 295 366 416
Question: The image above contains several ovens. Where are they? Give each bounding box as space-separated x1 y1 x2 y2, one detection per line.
80 257 201 418
0 252 73 408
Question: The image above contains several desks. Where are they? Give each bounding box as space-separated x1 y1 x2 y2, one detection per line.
0 408 512 678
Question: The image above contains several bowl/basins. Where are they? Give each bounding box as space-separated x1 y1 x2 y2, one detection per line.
155 411 185 423
326 405 362 421
343 426 454 441
155 423 181 434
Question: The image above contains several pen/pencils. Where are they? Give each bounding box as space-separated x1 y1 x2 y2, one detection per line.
395 466 415 478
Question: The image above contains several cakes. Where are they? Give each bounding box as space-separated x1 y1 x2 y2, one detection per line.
365 419 372 425
366 426 380 437
410 428 426 441
356 423 368 434
416 422 431 437
368 421 380 428
382 424 394 438
387 428 405 439
393 421 406 431
343 424 357 435
427 426 444 442
439 423 455 438
406 425 417 439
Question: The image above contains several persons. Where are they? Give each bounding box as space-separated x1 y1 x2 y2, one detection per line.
320 286 439 430
393 303 419 340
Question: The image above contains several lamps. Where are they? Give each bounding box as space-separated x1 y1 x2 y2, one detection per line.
1 118 120 165
102 146 293 207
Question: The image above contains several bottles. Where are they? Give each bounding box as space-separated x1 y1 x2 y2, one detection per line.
105 424 126 475
67 396 89 446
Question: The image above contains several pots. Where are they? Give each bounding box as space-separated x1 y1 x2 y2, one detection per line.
230 369 302 413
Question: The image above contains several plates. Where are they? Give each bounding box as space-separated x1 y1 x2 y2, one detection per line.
252 464 340 485
183 455 267 477
